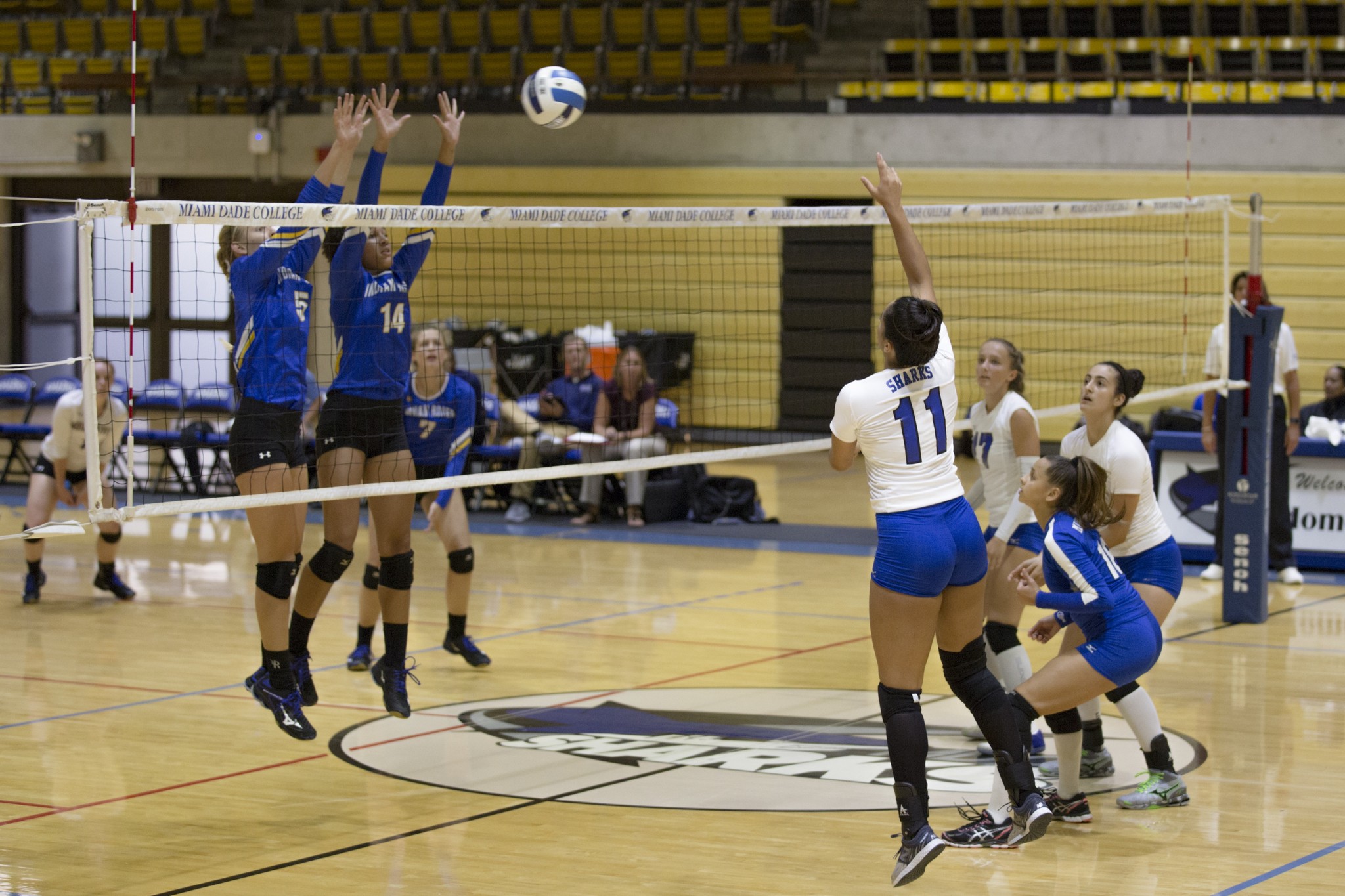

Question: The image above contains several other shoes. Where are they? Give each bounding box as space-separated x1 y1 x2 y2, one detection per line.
570 508 595 525
504 502 530 522
625 506 645 528
962 723 987 739
93 571 136 600
977 728 1046 756
1200 563 1224 580
22 570 46 605
1278 567 1304 584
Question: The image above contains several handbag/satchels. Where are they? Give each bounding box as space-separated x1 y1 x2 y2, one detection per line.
1148 405 1204 432
688 474 781 527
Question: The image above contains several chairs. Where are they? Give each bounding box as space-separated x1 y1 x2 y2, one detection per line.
0 374 255 493
468 390 682 520
835 0 1345 106
0 0 813 116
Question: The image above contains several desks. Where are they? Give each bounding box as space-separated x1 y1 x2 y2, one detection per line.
1144 429 1344 573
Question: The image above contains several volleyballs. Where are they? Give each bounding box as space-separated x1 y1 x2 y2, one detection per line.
520 66 588 129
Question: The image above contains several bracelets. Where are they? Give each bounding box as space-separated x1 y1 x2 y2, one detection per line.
1289 417 1301 426
1201 426 1214 434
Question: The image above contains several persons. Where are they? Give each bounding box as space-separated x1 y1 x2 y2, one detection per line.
286 84 465 718
939 455 1165 849
960 338 1046 761
21 358 136 605
216 91 371 743
1006 360 1190 811
1300 364 1345 433
829 150 1054 885
1198 270 1304 587
301 320 671 674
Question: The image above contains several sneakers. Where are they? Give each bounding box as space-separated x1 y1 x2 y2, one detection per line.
890 824 946 888
997 793 1053 846
1039 746 1115 778
292 648 318 706
442 629 491 668
243 665 317 742
941 796 1019 849
1038 787 1093 824
347 645 374 671
1116 768 1190 810
370 653 421 718
